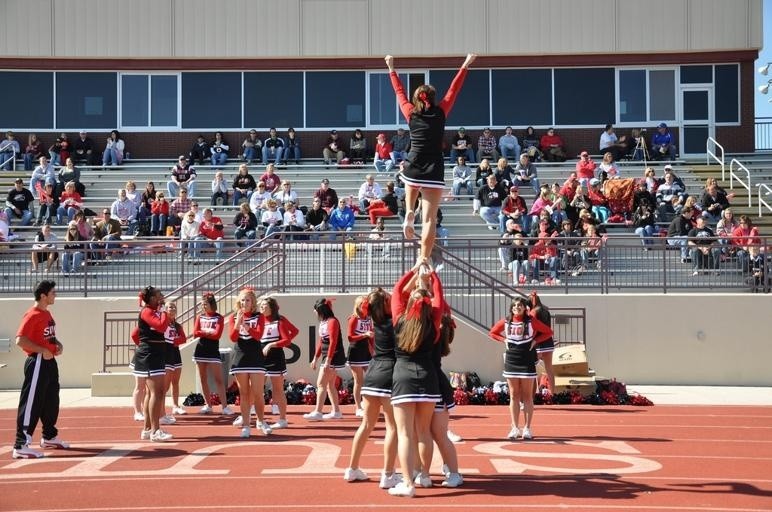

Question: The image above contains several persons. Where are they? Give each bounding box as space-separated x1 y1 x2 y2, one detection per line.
428 285 465 487
451 123 772 279
495 292 554 440
10 280 74 459
385 54 476 291
344 273 417 490
386 257 445 498
528 295 558 398
129 285 343 441
0 128 448 276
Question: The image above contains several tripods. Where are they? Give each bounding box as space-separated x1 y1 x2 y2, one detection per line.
632 135 650 169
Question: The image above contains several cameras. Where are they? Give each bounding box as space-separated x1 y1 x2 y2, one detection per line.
577 195 581 201
713 203 720 209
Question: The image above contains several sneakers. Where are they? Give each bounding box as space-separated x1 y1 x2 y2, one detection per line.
303 411 341 420
419 265 428 278
134 404 186 443
447 431 462 442
200 405 213 415
514 279 562 284
13 448 43 458
233 405 287 437
344 464 464 498
403 210 415 240
507 424 521 440
522 427 532 440
40 438 69 449
221 408 232 414
355 409 364 416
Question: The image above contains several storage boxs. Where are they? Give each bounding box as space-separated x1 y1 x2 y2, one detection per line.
553 344 588 375
554 371 595 396
